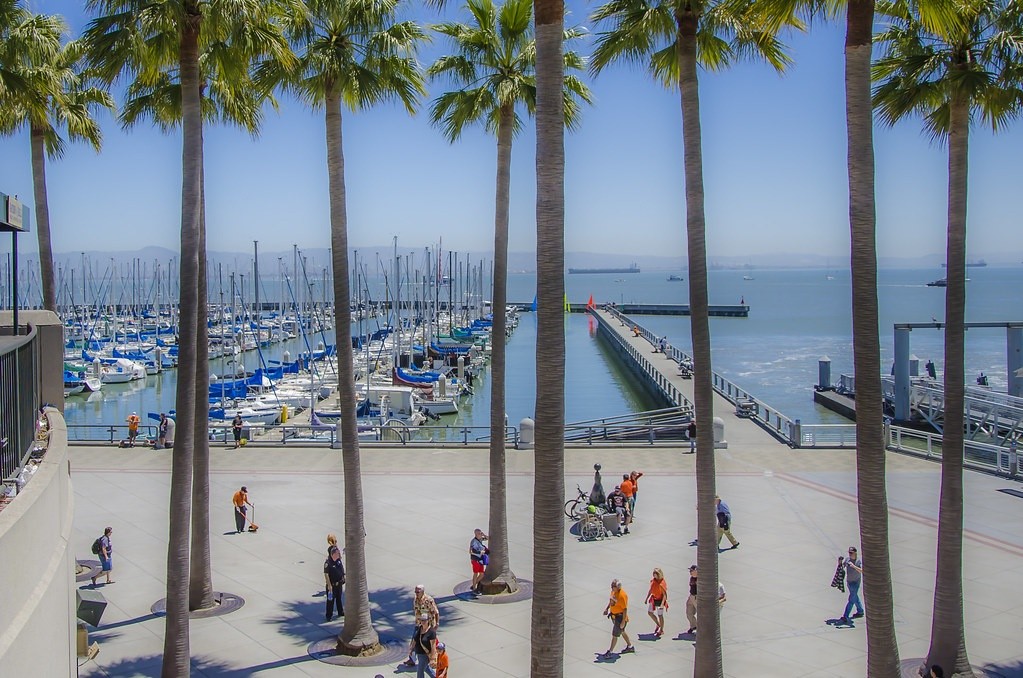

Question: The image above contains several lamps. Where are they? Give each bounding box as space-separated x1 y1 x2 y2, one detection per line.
76 588 107 628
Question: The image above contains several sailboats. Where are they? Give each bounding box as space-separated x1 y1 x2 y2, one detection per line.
0 237 526 437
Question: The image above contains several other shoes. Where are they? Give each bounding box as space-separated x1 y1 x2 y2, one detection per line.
841 611 863 622
327 612 344 622
691 451 694 453
470 586 482 596
234 446 237 449
106 580 115 584
731 542 739 549
604 625 697 659
617 528 631 535
91 576 96 586
404 658 415 666
238 528 245 533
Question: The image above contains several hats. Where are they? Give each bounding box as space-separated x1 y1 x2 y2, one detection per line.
241 486 247 493
132 412 136 416
614 485 620 489
688 565 698 571
848 547 857 553
623 474 629 479
436 642 445 649
690 418 695 423
417 613 431 620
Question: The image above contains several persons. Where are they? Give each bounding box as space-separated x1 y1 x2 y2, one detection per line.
431 642 448 678
233 486 248 532
91 527 113 585
835 546 864 625
411 613 437 678
602 579 635 659
232 414 243 449
125 412 140 447
323 546 346 620
211 429 217 440
686 418 696 453
718 582 726 612
714 495 739 549
645 568 668 636
156 413 167 449
685 565 698 633
606 471 643 535
469 528 488 595
660 336 666 353
404 584 439 666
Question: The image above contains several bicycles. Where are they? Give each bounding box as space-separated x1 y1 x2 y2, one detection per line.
565 485 606 541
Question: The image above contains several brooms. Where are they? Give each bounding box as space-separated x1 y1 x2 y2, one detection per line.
239 510 258 531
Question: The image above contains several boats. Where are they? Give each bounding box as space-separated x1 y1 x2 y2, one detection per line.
941 262 986 267
926 269 948 286
744 275 754 280
667 274 684 281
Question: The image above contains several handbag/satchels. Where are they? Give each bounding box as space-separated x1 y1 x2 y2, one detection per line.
484 545 490 554
654 599 662 606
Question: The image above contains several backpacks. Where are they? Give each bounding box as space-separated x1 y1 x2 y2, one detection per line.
91 535 109 554
